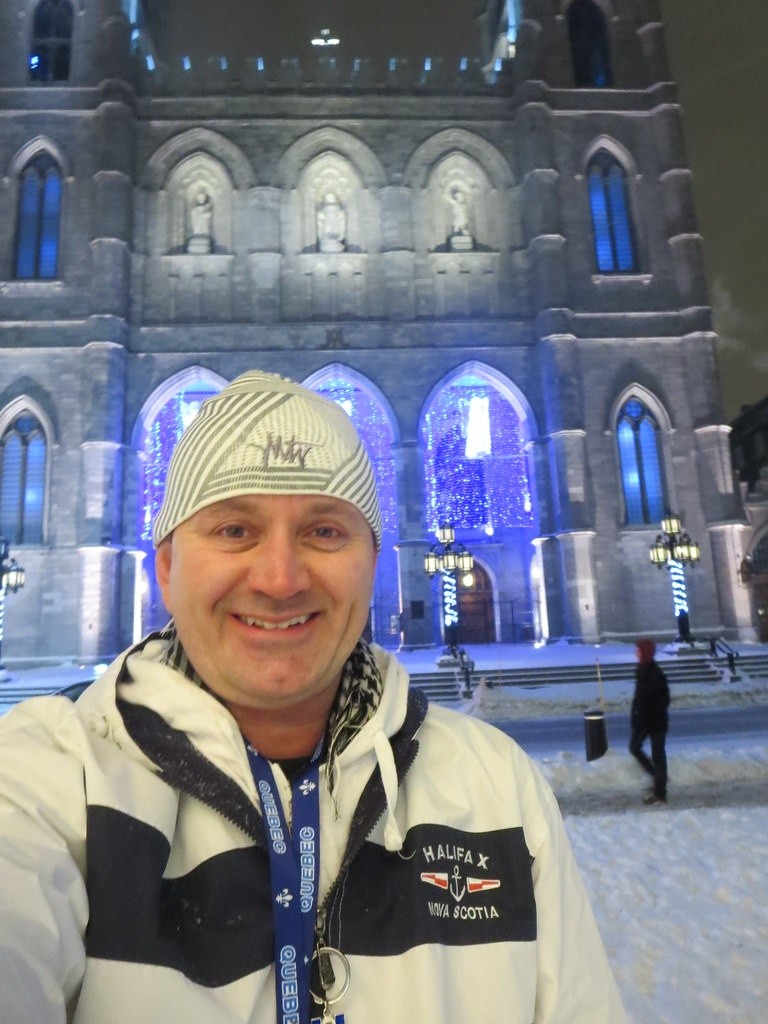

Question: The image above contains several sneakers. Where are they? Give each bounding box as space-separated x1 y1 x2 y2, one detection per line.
642 792 666 805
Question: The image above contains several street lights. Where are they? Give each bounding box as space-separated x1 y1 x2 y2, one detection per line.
0 538 26 683
424 521 474 665
649 508 710 655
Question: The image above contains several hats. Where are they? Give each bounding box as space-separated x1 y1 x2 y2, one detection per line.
152 371 383 557
636 639 657 655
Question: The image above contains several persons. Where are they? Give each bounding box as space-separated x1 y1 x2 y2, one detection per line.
0 369 629 1024
447 191 473 235
677 608 690 639
316 192 346 244
189 192 212 238
629 639 671 804
